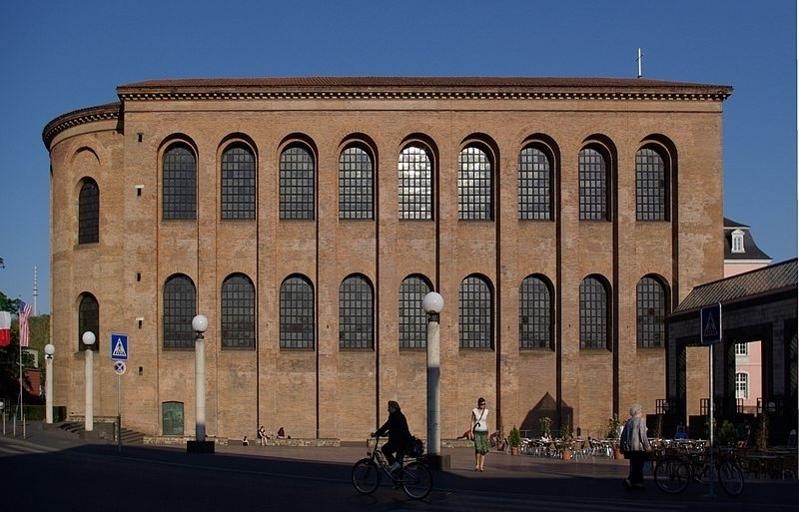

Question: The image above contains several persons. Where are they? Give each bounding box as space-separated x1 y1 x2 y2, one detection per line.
539 431 552 456
258 426 268 446
277 427 285 439
371 401 413 490
242 436 249 446
470 398 490 472
619 404 651 491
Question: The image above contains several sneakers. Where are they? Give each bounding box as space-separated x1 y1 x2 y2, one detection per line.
388 461 401 474
474 464 484 472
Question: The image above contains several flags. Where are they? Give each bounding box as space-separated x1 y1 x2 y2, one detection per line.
19 301 32 347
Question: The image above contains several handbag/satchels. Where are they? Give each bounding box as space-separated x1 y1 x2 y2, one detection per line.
464 429 476 441
404 436 424 457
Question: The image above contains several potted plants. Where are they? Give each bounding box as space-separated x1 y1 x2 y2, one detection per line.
606 413 625 460
557 426 575 461
507 423 523 456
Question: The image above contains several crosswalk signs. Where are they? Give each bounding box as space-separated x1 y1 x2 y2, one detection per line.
111 333 129 361
700 303 723 345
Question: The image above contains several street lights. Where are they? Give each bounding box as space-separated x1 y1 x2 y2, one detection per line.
420 291 445 456
42 342 57 424
190 312 210 442
79 330 100 430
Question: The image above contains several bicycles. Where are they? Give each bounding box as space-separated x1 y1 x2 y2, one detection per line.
351 432 433 500
652 446 746 498
